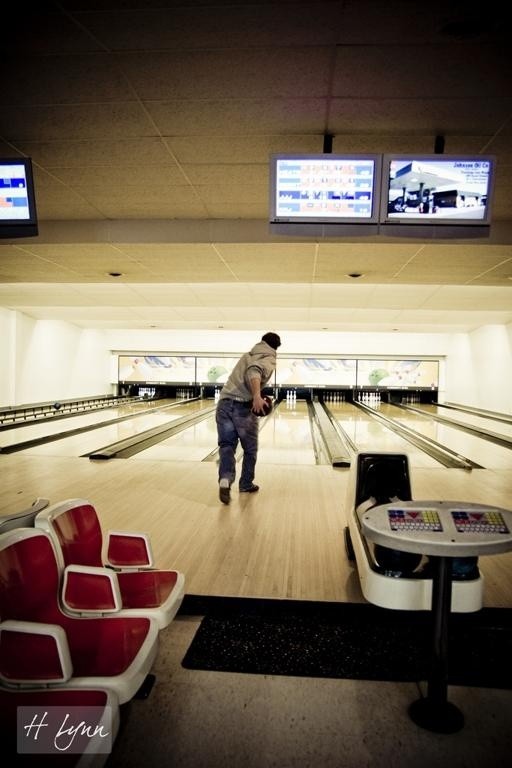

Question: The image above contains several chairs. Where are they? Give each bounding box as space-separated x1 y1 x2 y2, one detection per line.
0 497 187 768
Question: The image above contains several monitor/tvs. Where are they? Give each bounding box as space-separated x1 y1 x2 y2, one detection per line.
0 157 37 224
379 152 498 226
268 152 380 225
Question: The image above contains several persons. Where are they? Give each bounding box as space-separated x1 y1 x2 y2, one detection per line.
215 331 283 507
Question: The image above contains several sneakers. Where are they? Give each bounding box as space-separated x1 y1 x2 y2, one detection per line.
240 483 260 493
218 476 229 506
